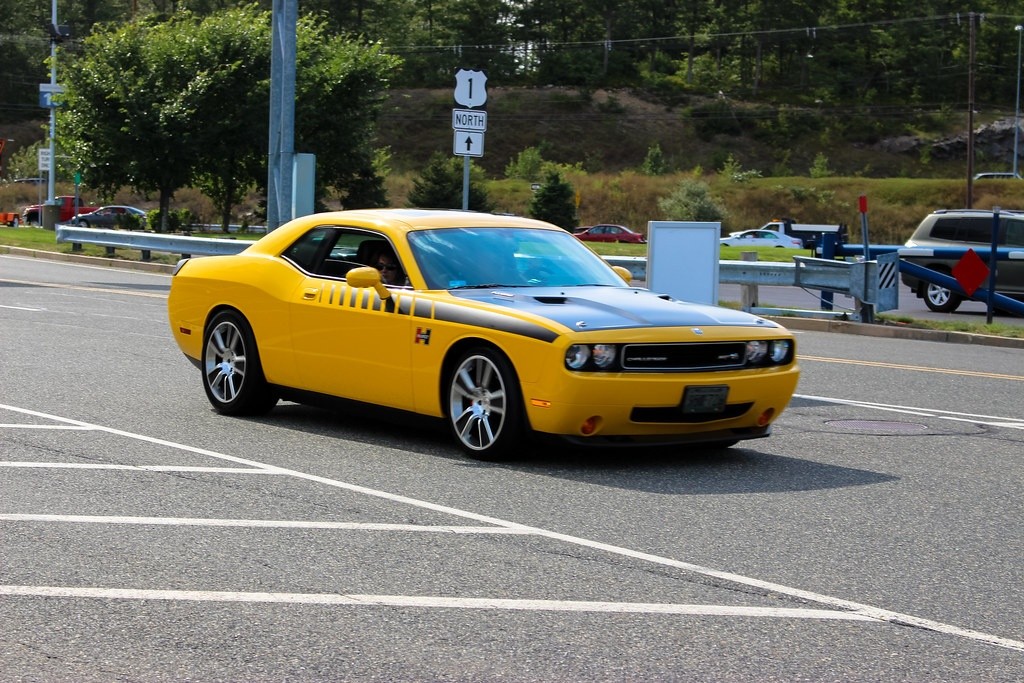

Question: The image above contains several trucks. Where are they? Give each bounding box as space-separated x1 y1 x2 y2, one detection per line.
760 219 848 256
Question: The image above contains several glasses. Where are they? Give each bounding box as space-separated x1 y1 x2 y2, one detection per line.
376 263 397 271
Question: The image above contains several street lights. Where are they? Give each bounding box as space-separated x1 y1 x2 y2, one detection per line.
1014 26 1023 180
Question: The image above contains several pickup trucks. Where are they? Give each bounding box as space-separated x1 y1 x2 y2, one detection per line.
24 196 110 224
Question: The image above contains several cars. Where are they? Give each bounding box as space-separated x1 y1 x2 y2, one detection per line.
974 172 1022 181
169 207 803 463
71 206 147 230
718 229 802 251
570 224 644 243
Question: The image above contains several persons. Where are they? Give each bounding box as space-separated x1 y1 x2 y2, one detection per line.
375 255 397 285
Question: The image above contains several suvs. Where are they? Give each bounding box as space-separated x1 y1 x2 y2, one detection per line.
900 209 1024 312
